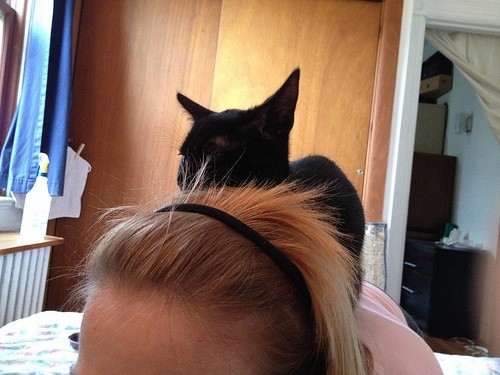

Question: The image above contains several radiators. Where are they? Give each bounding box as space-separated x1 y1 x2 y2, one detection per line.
0 246 51 328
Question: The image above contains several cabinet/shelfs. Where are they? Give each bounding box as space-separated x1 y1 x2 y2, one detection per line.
399 239 479 339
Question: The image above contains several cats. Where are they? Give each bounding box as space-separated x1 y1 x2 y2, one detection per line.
152 64 365 313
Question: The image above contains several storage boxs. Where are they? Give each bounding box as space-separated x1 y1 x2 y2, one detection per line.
421 51 453 95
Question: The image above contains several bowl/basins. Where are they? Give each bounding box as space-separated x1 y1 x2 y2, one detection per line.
68 333 79 350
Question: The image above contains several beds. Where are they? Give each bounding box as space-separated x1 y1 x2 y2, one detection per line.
0 311 500 375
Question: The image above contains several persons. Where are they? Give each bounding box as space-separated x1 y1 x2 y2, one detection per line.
69 185 445 375
